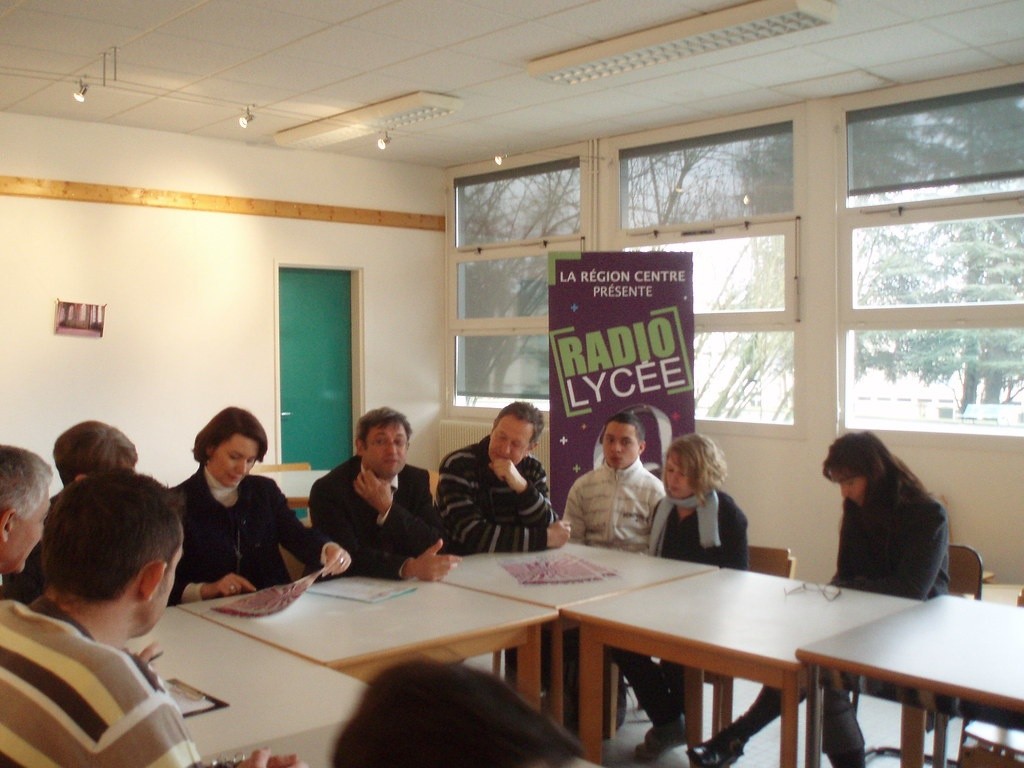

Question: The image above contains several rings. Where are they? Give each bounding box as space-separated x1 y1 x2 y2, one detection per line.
337 557 344 564
229 585 236 591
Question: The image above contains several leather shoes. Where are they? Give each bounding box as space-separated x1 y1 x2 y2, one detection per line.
687 728 745 768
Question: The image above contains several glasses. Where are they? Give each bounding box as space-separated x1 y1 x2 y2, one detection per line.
365 438 409 450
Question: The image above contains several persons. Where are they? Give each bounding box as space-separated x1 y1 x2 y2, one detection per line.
166 406 351 607
561 411 667 741
0 444 53 606
1 420 138 607
684 428 951 768
610 431 748 762
433 400 571 694
307 406 463 584
0 470 307 768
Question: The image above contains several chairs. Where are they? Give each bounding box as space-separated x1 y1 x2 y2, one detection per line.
947 546 982 598
704 545 795 737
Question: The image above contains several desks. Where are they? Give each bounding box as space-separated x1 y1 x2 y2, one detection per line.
433 543 712 741
117 608 376 768
177 575 558 711
559 566 925 768
793 595 1024 767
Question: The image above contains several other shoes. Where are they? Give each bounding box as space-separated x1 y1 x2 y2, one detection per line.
634 714 685 762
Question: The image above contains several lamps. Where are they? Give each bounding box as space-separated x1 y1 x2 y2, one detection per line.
74 82 89 103
495 153 508 164
377 130 391 152
239 106 256 129
